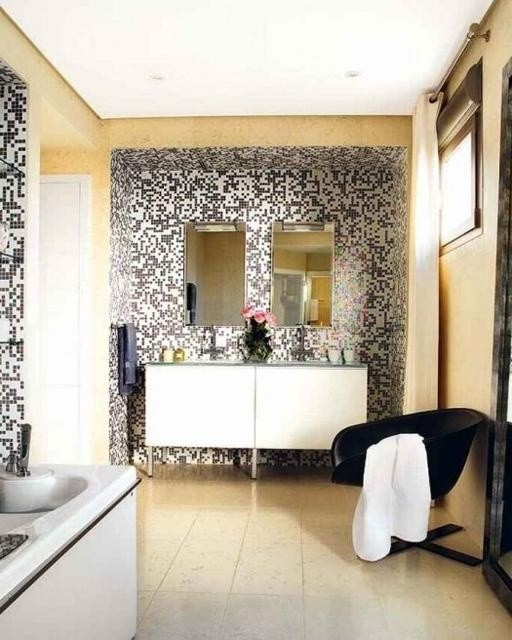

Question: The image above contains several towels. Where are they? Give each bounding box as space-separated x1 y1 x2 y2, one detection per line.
118 324 137 396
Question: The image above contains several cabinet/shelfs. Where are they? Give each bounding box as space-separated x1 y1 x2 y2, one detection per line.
145 364 367 479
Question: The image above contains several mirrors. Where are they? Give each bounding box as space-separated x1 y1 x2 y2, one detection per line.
186 221 245 325
270 222 333 326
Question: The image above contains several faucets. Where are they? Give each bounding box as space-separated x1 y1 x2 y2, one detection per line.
293 322 314 361
205 322 226 358
6 422 34 478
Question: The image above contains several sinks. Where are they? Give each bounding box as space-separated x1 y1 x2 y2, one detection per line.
179 361 248 367
0 471 91 536
270 363 337 368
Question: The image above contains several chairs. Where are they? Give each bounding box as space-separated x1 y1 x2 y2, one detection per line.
331 408 484 566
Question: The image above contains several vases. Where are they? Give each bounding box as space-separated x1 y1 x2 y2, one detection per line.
242 343 271 362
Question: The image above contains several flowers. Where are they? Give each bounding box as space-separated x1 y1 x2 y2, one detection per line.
239 302 277 361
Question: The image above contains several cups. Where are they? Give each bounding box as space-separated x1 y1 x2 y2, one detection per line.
327 350 343 365
344 349 356 365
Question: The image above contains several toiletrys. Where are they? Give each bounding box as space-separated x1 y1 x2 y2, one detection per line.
174 346 186 364
161 344 174 365
